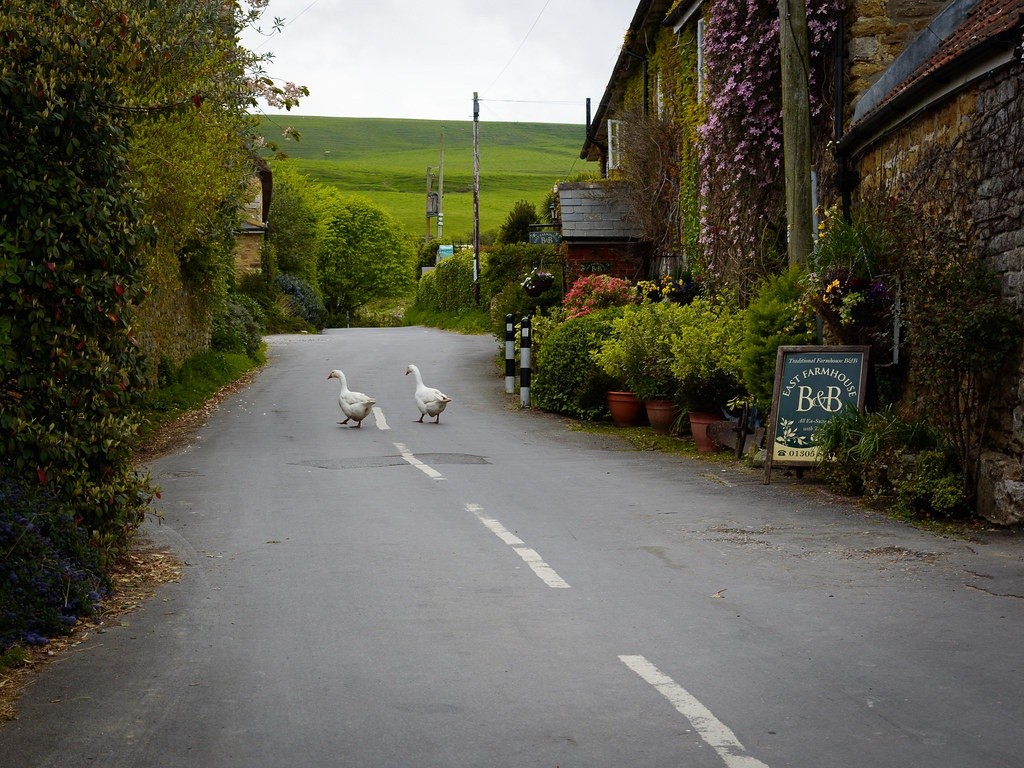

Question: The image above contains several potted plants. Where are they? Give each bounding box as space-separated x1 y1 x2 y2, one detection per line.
587 288 746 452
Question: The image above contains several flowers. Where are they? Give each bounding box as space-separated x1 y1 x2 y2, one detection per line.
822 269 891 327
521 268 555 288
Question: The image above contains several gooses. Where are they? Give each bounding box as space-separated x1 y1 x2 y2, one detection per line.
328 370 376 428
406 365 451 425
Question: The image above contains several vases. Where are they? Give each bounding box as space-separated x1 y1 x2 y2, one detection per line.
525 279 554 297
